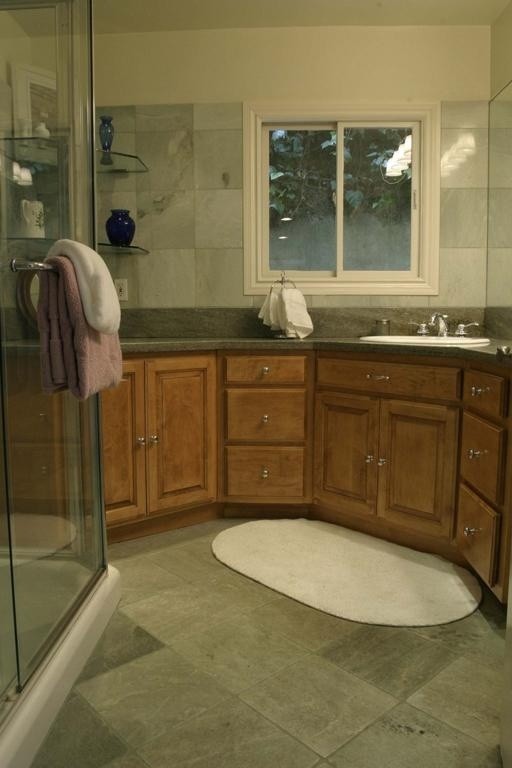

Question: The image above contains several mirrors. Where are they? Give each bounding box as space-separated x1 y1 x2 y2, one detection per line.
16 256 44 333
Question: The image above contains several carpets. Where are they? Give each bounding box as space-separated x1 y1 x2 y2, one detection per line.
211 519 481 628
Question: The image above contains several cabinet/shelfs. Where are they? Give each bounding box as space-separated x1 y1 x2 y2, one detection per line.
314 354 468 557
455 353 512 602
217 354 317 522
103 353 216 546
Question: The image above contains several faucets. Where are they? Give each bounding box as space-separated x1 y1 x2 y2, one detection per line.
429 312 450 336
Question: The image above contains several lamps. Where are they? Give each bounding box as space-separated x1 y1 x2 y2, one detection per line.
441 134 477 177
386 134 412 177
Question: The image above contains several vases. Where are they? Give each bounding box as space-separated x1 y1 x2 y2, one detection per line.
35 120 50 149
98 115 115 164
105 209 134 245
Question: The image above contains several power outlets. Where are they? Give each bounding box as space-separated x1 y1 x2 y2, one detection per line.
114 278 128 302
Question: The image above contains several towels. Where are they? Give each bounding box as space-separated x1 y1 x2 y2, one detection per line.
36 237 124 401
258 284 314 339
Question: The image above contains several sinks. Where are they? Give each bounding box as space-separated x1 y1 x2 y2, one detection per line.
360 335 489 344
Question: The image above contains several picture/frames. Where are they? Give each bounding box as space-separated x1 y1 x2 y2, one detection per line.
9 61 59 167
